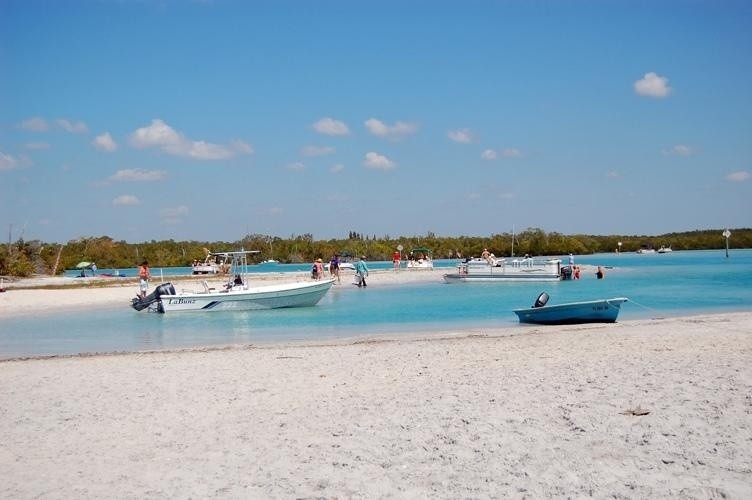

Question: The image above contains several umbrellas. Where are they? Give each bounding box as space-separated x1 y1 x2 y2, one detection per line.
76 261 91 272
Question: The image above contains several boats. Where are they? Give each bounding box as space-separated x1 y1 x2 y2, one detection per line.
160 233 355 313
407 249 433 268
94 273 126 278
636 245 672 253
442 256 561 283
511 298 628 323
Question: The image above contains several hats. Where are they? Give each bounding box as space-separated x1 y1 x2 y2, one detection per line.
316 258 322 263
361 256 367 259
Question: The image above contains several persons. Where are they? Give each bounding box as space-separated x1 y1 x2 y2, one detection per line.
595 266 603 280
392 251 401 273
312 258 324 281
138 261 151 297
328 254 342 285
572 265 580 280
91 262 97 277
356 255 369 287
576 266 580 273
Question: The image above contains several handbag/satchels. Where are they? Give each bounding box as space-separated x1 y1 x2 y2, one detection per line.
313 265 317 272
